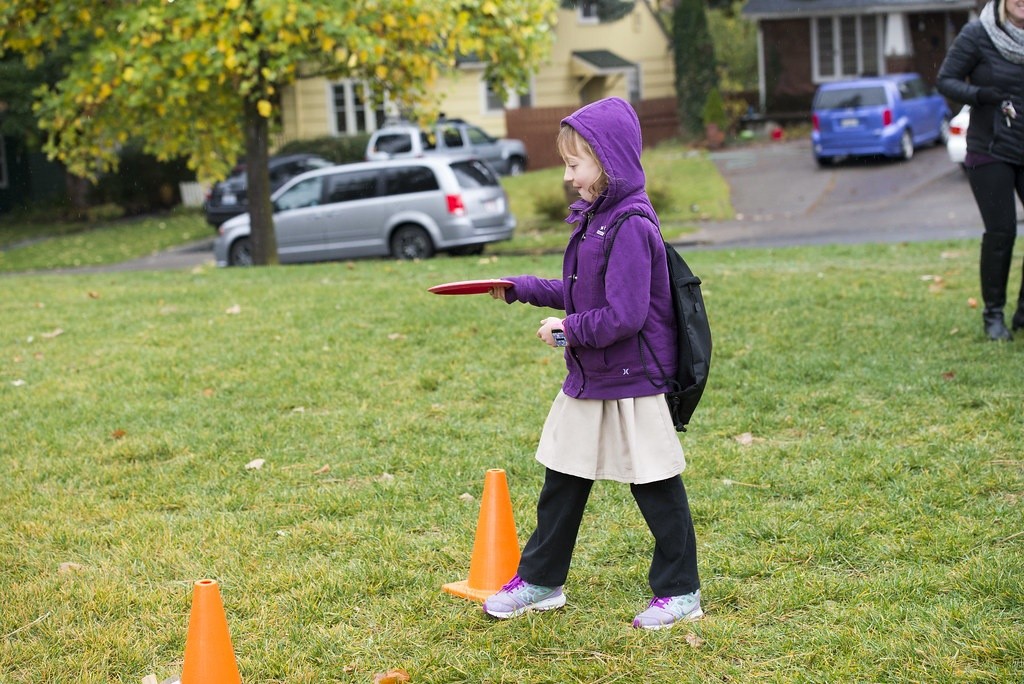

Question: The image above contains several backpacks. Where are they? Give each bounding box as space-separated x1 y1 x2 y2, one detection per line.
602 212 712 426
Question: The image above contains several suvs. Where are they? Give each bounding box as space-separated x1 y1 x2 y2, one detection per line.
364 119 531 175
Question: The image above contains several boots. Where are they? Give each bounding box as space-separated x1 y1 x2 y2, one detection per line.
1012 257 1024 331
980 233 1016 342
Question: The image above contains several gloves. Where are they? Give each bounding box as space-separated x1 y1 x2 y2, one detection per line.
977 85 1010 105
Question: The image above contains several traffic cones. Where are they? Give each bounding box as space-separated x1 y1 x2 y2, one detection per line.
441 469 522 605
178 578 242 684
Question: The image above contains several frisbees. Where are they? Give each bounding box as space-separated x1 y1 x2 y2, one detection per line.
427 278 517 295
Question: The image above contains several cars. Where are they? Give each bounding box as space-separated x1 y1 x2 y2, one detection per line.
947 105 974 163
203 152 336 229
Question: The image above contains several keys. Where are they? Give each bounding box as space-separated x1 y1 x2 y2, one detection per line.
1000 99 1016 128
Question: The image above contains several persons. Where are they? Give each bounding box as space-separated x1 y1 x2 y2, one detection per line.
935 0 1024 343
482 96 704 631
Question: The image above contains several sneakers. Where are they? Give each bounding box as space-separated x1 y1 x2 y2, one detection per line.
633 589 705 632
483 574 566 619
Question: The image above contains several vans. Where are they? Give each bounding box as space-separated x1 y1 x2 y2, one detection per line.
213 160 515 269
812 75 951 168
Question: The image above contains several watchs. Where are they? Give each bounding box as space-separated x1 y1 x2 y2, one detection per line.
551 318 568 347
